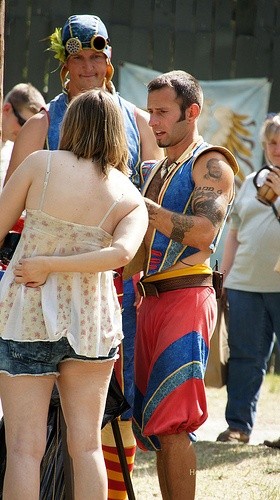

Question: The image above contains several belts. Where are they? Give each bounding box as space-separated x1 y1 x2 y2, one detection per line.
135 273 212 298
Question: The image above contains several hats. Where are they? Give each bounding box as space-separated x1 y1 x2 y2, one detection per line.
49 13 113 66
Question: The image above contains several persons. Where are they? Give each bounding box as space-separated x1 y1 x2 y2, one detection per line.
0 83 46 191
216 112 280 448
0 15 165 500
131 70 240 500
0 89 149 500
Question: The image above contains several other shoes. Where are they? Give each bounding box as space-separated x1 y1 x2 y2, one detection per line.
216 425 250 443
263 436 280 450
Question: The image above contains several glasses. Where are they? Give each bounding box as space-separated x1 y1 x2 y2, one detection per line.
8 99 27 127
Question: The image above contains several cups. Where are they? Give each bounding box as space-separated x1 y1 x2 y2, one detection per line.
252 165 280 206
